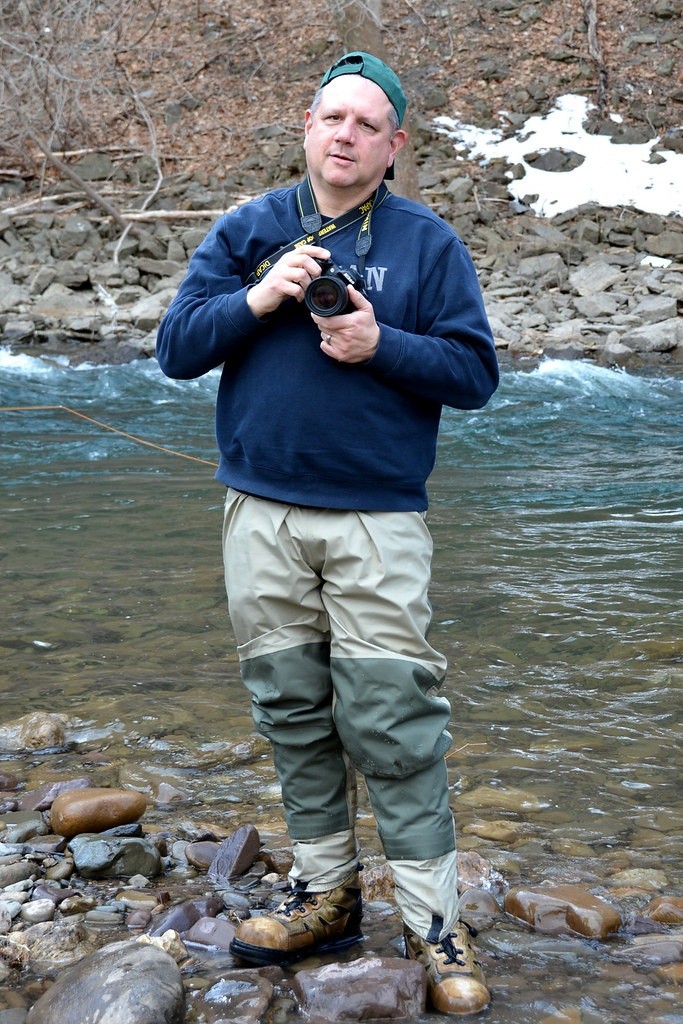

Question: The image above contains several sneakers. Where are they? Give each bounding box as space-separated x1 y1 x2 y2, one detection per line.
403 925 490 1014
229 871 364 964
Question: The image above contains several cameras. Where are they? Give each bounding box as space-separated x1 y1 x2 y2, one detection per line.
291 256 369 318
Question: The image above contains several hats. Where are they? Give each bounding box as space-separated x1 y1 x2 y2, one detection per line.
320 51 407 181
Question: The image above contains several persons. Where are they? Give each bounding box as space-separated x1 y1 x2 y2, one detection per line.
158 50 501 1019
